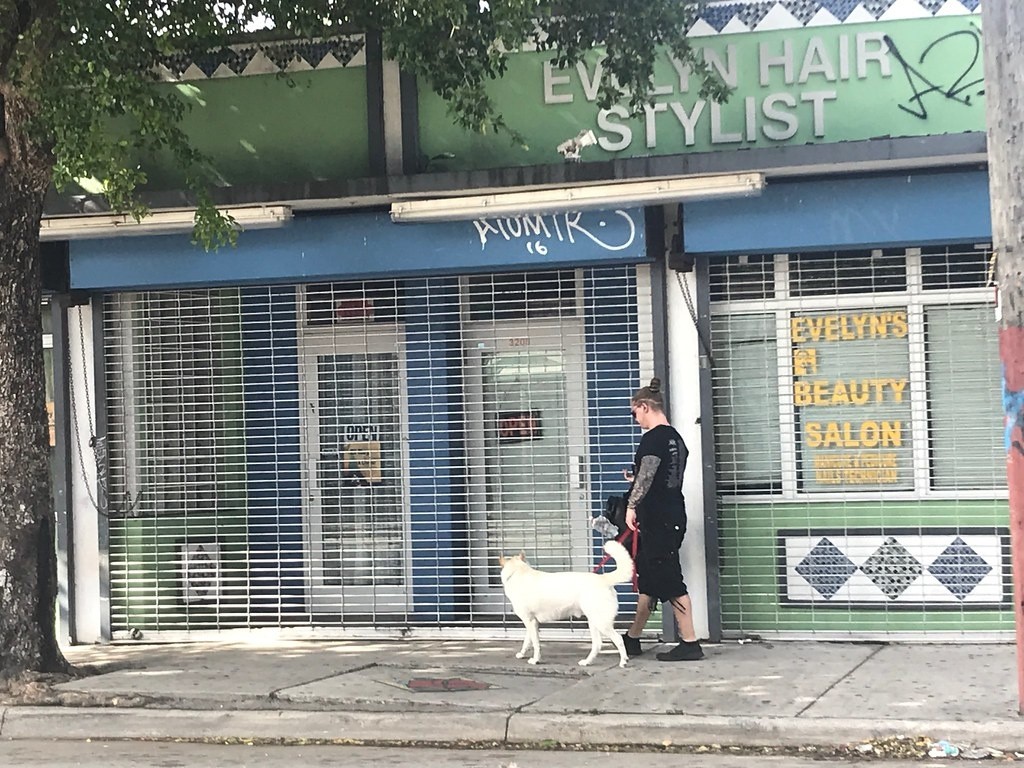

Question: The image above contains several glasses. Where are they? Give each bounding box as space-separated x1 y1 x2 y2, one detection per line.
630 405 641 418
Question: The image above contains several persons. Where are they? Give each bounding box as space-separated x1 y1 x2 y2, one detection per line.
620 378 705 662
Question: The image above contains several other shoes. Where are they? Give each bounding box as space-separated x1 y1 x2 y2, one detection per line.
656 641 703 661
620 632 642 655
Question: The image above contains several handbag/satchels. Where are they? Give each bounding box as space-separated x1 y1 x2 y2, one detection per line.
605 496 632 543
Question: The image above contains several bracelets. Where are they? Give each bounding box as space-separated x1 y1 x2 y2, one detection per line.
627 505 636 509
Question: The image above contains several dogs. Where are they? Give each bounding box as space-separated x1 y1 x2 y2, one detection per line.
498 538 637 668
